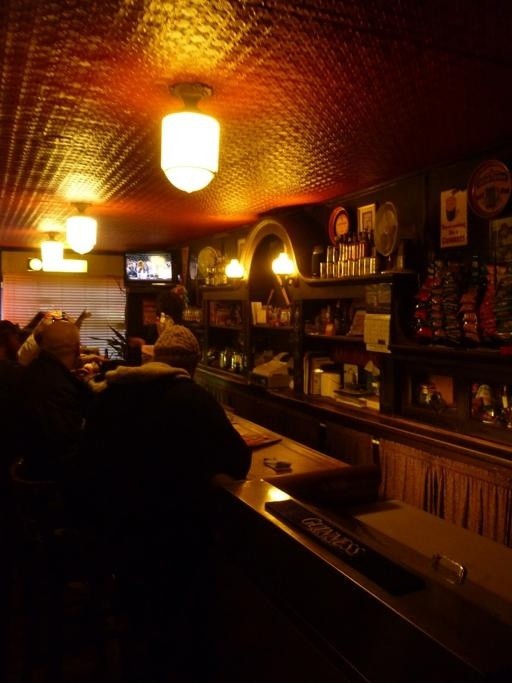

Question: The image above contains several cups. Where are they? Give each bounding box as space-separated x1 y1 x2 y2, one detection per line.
231 354 243 370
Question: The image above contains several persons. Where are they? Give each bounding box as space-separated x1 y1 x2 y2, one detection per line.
91 326 253 615
0 309 96 614
126 259 173 281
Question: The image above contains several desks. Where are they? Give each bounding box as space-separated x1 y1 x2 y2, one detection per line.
165 420 491 541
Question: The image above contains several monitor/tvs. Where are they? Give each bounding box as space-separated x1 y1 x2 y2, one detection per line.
123 250 178 285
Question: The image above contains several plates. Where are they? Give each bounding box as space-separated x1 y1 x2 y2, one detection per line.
371 201 396 257
328 205 349 243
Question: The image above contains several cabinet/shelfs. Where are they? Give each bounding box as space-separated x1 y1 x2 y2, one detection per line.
127 293 179 375
180 173 413 418
414 170 512 429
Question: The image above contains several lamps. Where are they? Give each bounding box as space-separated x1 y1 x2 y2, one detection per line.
158 75 227 198
62 196 104 256
38 228 65 272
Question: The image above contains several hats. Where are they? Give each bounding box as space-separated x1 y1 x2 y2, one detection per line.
153 324 202 365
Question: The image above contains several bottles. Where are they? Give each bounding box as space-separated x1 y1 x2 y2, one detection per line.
333 226 373 246
419 383 445 415
